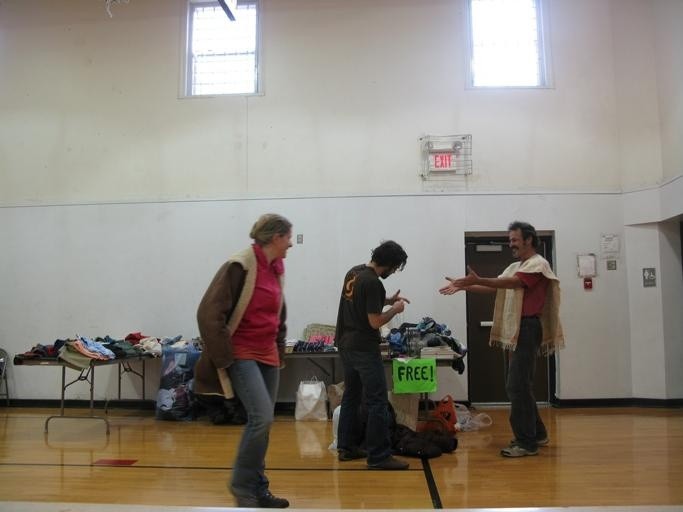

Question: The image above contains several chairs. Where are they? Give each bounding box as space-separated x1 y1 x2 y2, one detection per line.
0 344 12 415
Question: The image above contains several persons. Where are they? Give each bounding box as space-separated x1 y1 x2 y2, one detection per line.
192 215 293 507
334 241 410 471
439 223 550 457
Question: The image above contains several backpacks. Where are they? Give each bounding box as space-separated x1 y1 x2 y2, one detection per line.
207 397 246 425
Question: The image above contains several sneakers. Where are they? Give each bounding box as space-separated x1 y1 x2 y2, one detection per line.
338 448 368 461
228 483 259 508
501 445 538 457
367 456 409 471
511 433 550 446
260 489 290 508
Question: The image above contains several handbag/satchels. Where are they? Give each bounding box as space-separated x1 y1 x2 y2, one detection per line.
386 389 458 458
294 376 329 422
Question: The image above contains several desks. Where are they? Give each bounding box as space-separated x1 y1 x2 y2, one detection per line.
12 349 157 431
380 350 472 443
192 347 338 425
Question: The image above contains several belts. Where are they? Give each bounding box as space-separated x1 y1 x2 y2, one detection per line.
523 315 539 320
